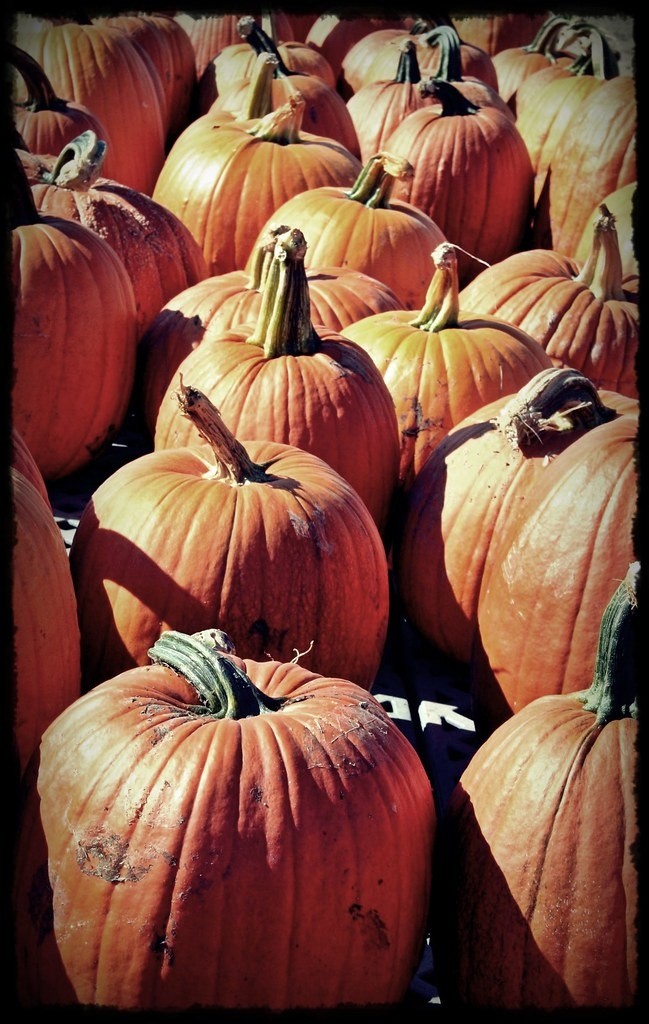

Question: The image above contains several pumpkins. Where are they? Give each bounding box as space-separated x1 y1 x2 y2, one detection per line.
13 10 638 1008
